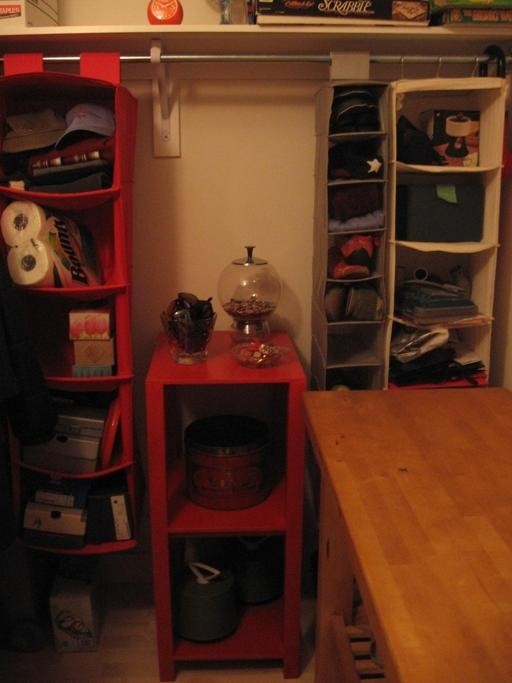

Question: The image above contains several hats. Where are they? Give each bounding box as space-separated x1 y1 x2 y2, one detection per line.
335 85 382 132
56 102 116 145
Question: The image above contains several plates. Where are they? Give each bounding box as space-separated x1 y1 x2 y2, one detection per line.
229 341 278 370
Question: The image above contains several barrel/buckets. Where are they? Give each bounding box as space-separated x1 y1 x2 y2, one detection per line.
183 415 274 511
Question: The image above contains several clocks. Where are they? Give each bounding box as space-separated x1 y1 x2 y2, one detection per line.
146 0 184 25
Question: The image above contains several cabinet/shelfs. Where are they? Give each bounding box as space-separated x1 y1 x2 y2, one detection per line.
390 76 504 386
0 71 140 561
304 386 512 680
314 77 389 387
147 324 309 682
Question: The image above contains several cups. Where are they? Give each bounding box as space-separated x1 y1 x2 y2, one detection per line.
158 311 216 366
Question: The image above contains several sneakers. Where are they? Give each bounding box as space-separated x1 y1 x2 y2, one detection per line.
326 233 383 321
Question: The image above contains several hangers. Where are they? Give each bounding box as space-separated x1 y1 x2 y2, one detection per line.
421 54 444 103
393 54 404 112
467 57 483 78
